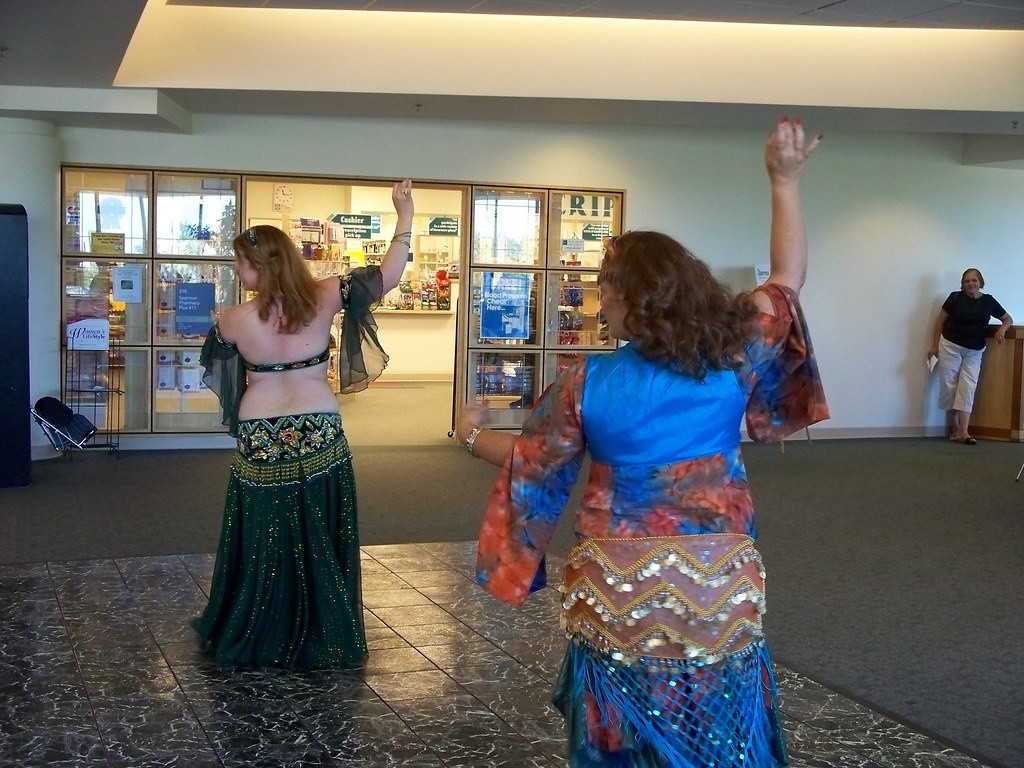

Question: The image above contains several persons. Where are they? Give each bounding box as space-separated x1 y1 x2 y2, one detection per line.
928 268 1013 444
192 179 414 670
455 114 829 768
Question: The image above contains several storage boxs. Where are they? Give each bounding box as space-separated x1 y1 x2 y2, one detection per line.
156 286 209 392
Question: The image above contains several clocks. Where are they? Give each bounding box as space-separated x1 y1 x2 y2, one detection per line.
272 184 295 204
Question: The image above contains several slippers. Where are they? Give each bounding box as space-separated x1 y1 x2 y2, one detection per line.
957 435 977 444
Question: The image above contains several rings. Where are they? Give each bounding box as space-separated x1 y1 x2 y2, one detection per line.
402 192 406 194
1000 337 1002 338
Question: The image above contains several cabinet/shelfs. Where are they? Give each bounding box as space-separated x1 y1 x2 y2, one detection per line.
65 334 125 462
558 279 610 345
362 239 388 268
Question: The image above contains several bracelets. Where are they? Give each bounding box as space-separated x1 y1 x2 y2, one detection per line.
391 232 411 248
465 426 490 457
